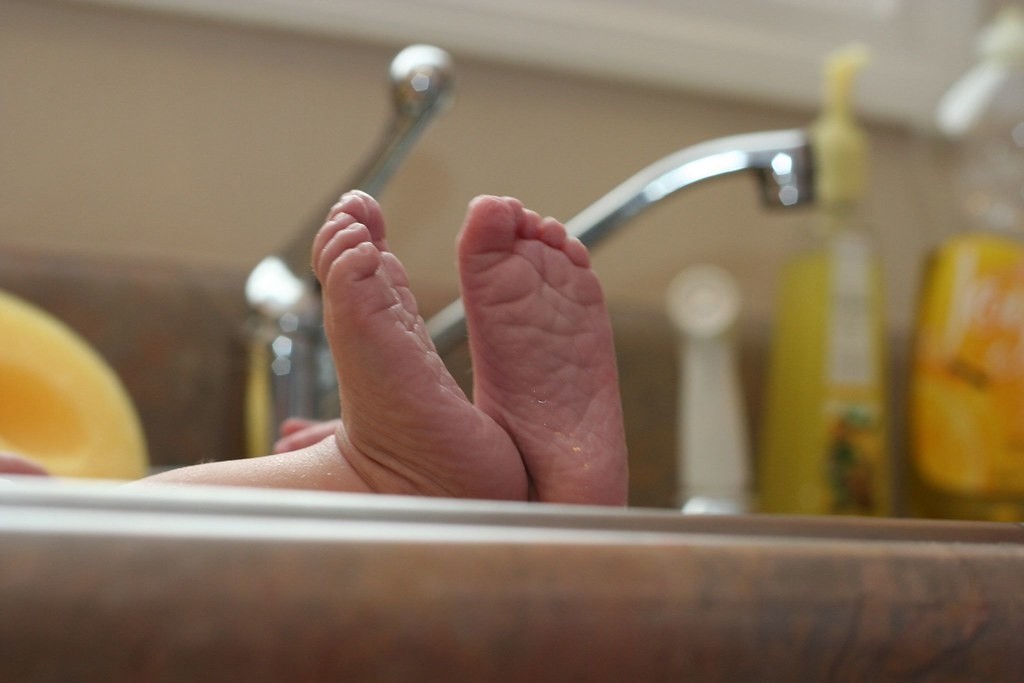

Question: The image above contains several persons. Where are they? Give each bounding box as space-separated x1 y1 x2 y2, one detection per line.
0 189 631 509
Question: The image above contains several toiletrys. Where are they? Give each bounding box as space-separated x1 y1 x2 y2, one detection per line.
756 38 898 518
908 231 1024 522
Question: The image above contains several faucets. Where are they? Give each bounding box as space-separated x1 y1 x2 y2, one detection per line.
423 125 822 359
